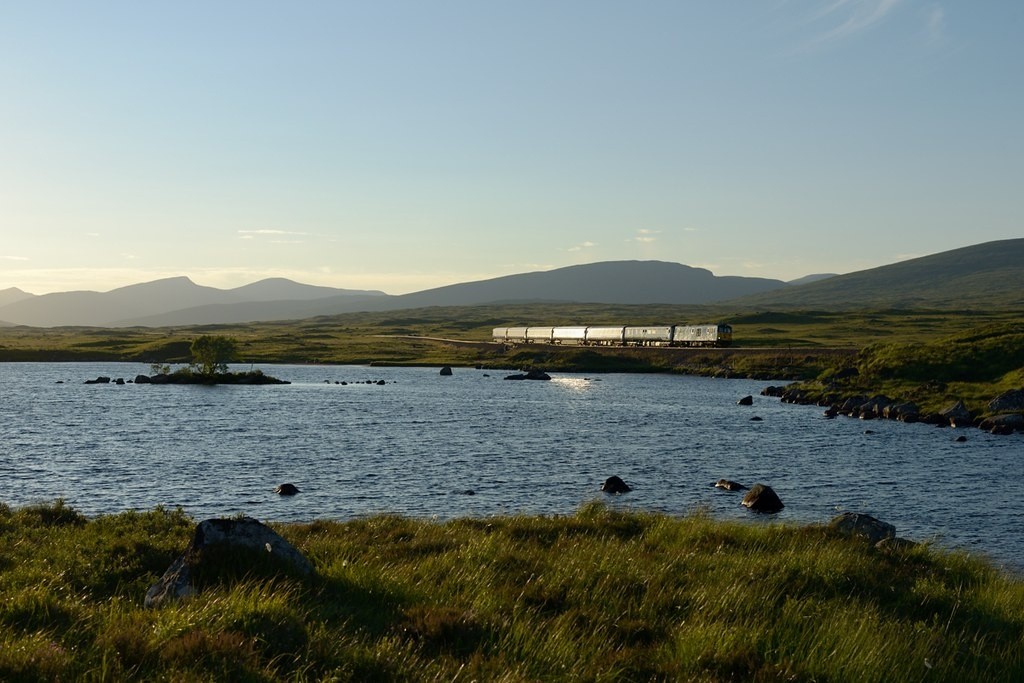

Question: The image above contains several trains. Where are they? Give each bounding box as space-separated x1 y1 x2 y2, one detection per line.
492 321 734 348
